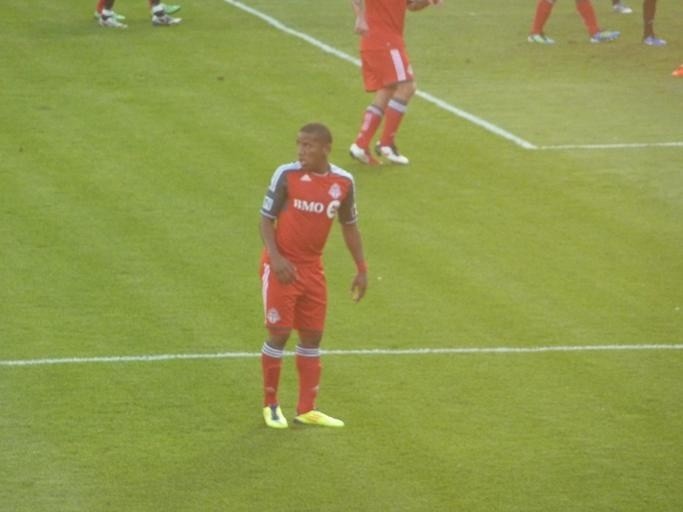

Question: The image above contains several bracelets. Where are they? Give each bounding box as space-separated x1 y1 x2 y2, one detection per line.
354 261 367 273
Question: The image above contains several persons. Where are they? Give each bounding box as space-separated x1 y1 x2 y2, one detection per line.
93 0 179 20
256 125 368 430
98 0 181 28
526 1 622 47
347 1 441 168
609 0 669 47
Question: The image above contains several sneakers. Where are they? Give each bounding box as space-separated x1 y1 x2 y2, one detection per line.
95 10 127 29
152 3 181 26
615 5 632 13
590 30 619 42
528 33 553 44
643 37 665 47
295 410 343 426
263 403 288 428
350 141 408 167
